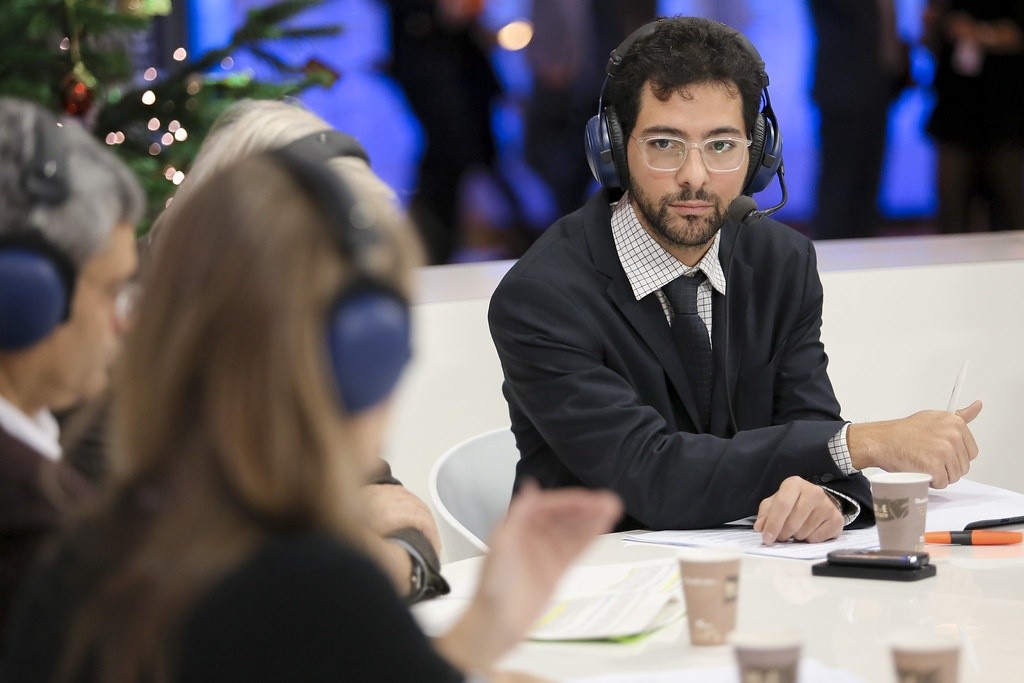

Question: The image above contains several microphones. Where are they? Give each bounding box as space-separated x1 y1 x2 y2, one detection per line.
729 159 788 226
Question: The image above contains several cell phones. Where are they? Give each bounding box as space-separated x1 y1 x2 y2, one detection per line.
828 549 929 569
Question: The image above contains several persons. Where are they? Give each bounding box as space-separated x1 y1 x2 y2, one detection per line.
1 86 621 682
520 0 660 220
376 1 554 266
919 0 1024 235
804 0 914 237
488 15 986 552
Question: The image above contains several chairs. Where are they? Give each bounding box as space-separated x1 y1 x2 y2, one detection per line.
425 424 521 561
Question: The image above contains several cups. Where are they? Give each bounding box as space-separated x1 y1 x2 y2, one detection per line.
870 472 932 552
893 641 962 683
734 634 799 683
678 549 742 645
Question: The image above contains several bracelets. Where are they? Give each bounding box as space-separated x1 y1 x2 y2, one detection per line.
823 489 845 514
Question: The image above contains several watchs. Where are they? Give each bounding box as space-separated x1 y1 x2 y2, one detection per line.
409 554 425 596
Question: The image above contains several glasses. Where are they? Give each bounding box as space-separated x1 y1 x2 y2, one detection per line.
629 129 753 173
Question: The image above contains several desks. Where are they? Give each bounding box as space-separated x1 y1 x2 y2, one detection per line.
407 464 1024 683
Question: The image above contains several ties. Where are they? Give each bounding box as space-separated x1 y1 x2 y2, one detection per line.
656 273 716 432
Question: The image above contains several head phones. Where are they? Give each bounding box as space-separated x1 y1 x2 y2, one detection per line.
0 104 74 352
585 16 783 198
250 146 415 416
